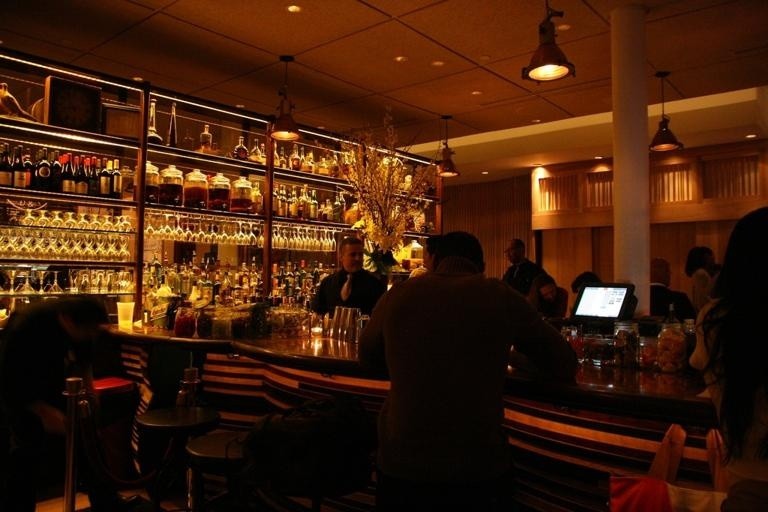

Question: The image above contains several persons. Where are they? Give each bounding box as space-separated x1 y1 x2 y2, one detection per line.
680 245 716 302
700 208 768 309
358 230 578 512
529 275 568 316
1 293 145 512
2 294 110 471
693 310 768 489
571 272 601 293
650 257 698 318
503 239 547 297
312 236 384 315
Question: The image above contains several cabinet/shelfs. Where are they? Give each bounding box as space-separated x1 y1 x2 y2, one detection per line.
0 52 444 335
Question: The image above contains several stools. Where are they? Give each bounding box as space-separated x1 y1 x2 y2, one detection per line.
186 435 253 512
135 405 223 512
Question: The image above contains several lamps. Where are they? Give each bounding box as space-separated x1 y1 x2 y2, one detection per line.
520 18 577 83
647 118 683 153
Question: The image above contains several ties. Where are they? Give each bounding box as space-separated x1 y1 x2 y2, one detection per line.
340 273 352 301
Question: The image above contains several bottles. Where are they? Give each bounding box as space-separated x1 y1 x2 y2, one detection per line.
293 262 301 287
222 272 233 299
200 124 212 154
231 177 253 212
277 186 286 216
322 199 333 221
168 270 178 295
249 140 262 162
23 149 33 189
333 196 342 223
239 263 250 285
318 158 328 175
38 148 51 190
178 266 185 277
12 145 26 188
291 146 300 169
226 264 233 284
75 156 89 195
154 252 162 287
111 160 123 198
299 262 307 289
234 137 250 159
271 261 281 287
183 169 208 209
285 262 294 288
182 266 190 294
272 182 278 217
242 274 249 301
250 264 257 284
62 153 76 192
202 271 213 304
288 187 298 217
149 265 158 289
168 106 178 147
51 151 62 193
147 99 163 144
160 165 184 206
145 161 159 203
0 143 13 186
319 263 324 280
213 271 222 304
252 182 263 214
280 147 287 169
302 156 312 173
310 190 318 219
234 273 242 299
279 266 285 280
304 270 314 290
98 158 111 197
312 261 320 286
299 184 310 217
319 203 325 220
89 157 100 196
209 173 231 211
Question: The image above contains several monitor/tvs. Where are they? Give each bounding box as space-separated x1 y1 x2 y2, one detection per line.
570 280 637 336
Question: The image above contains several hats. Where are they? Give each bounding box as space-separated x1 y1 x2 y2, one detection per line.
504 239 524 252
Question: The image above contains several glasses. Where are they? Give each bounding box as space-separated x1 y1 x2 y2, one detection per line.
348 251 363 256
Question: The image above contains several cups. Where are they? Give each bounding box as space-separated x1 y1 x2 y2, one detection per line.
20 209 132 233
272 225 336 252
2 269 21 294
17 271 34 294
47 271 64 294
116 302 135 334
144 209 265 249
0 237 132 262
33 270 50 294
